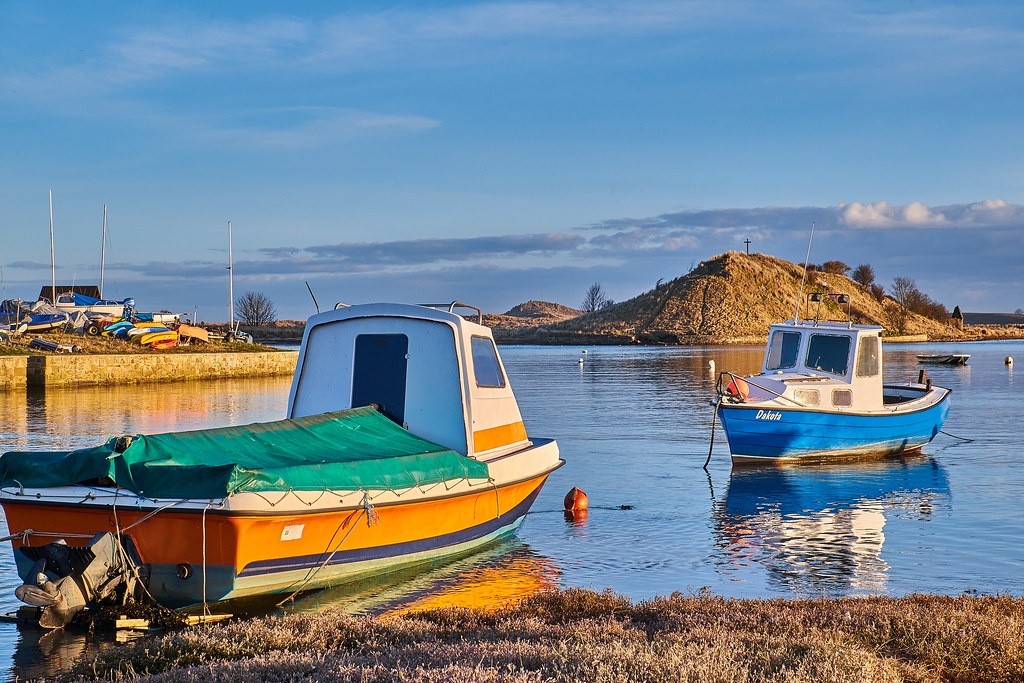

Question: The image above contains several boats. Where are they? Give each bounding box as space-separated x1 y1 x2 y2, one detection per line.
916 353 972 366
0 289 253 354
0 300 569 623
710 222 954 460
